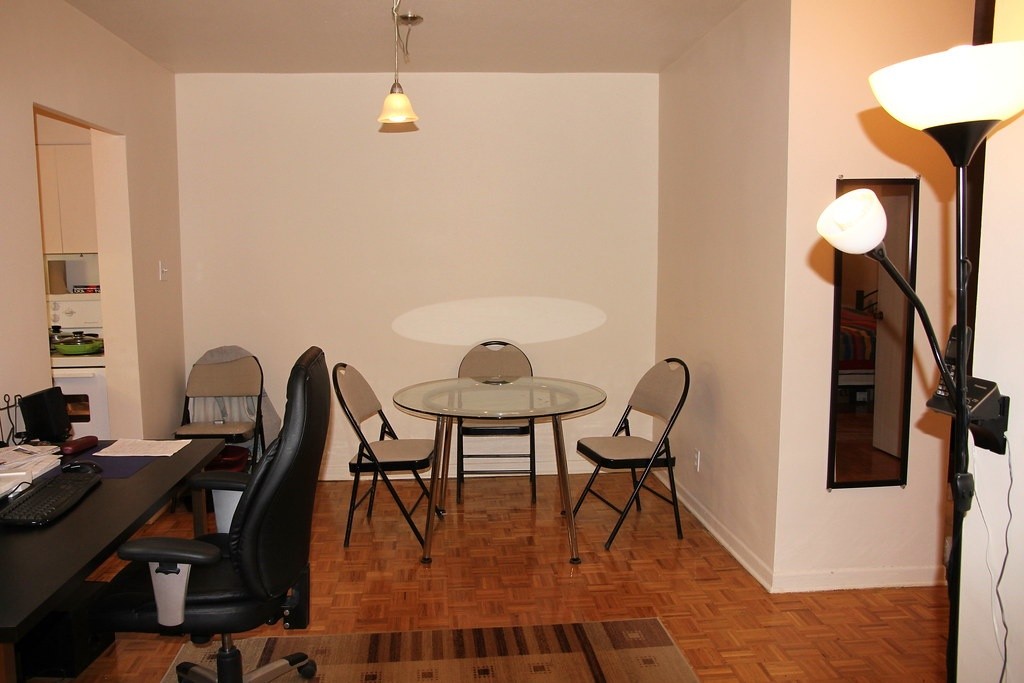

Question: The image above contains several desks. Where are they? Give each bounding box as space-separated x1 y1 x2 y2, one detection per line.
393 374 607 564
0 438 227 683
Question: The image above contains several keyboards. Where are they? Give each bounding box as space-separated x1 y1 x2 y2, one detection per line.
0 473 102 525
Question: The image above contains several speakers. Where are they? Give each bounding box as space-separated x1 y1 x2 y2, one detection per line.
18 387 71 444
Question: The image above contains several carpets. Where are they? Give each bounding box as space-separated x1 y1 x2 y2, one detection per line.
164 616 703 683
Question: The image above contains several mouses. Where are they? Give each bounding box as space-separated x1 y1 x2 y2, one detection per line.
61 460 103 474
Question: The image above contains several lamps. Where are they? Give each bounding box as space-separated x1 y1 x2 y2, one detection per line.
815 41 1024 683
376 0 424 124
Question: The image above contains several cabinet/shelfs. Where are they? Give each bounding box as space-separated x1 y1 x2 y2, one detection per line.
36 143 99 254
43 253 104 328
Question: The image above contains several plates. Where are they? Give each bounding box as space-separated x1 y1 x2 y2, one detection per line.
49 333 99 340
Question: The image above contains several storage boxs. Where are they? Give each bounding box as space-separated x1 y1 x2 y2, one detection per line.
13 581 116 679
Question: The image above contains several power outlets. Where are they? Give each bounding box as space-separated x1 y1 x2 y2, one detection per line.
159 258 169 282
693 448 701 473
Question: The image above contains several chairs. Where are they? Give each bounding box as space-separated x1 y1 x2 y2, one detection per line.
458 340 538 504
168 345 266 515
332 362 444 549
103 346 332 683
575 357 691 550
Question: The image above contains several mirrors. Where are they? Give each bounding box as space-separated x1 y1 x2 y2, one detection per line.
823 178 921 491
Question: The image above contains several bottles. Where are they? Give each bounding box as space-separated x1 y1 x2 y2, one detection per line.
49 325 63 353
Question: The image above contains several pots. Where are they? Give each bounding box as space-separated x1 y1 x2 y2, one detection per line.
53 330 102 355
